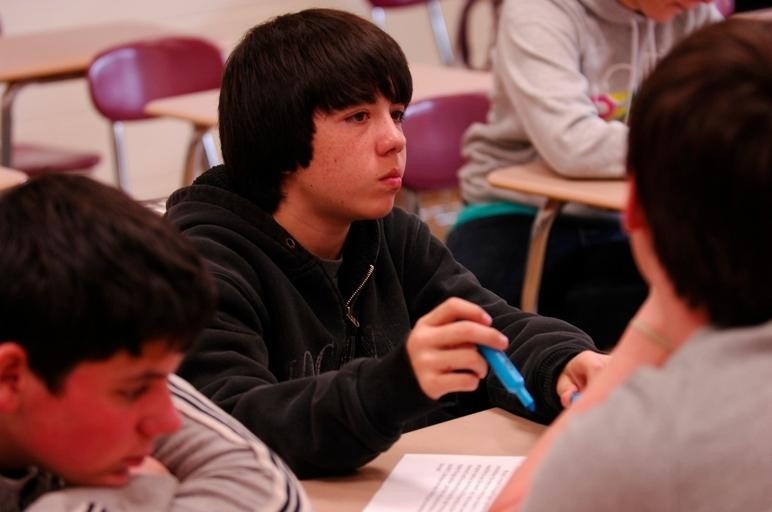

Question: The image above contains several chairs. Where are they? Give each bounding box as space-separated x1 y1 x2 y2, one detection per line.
2 0 494 247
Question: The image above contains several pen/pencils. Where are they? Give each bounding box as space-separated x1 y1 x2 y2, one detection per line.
479 343 538 414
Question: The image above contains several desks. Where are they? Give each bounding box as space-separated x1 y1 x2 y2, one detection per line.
466 160 629 316
302 404 552 511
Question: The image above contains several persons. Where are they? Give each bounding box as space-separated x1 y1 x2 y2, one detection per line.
443 2 729 353
0 170 313 512
158 5 611 482
483 8 772 510
